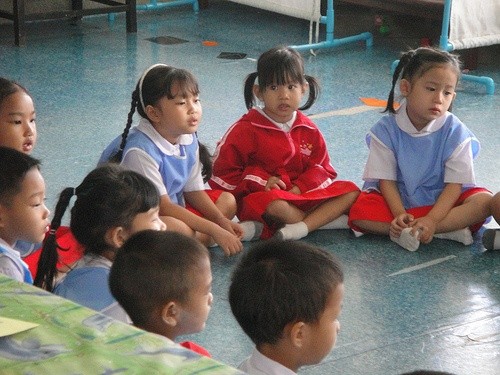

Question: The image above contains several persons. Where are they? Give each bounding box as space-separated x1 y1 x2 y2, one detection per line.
481 191 500 252
0 75 344 373
347 46 494 251
96 63 246 257
206 46 361 242
